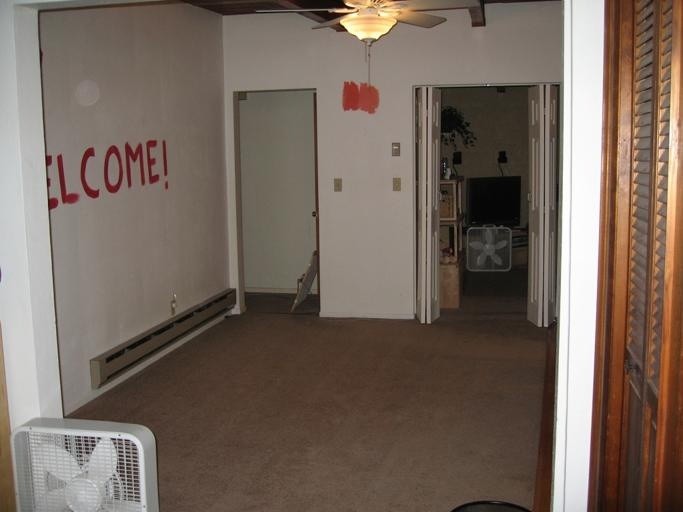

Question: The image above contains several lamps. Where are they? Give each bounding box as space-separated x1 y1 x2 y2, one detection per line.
335 0 403 92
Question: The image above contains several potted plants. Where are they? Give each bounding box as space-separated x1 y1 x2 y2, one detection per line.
441 107 478 150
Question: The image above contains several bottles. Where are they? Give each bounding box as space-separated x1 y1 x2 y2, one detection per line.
441 157 448 175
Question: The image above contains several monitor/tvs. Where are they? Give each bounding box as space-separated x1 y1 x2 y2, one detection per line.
466 175 521 227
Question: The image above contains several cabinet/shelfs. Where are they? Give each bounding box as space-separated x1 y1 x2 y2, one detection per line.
440 179 463 309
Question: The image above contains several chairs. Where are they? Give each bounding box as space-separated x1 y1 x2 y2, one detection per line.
451 501 531 512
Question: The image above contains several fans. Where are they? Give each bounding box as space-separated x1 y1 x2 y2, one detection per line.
467 223 512 272
255 0 483 30
11 416 159 512
